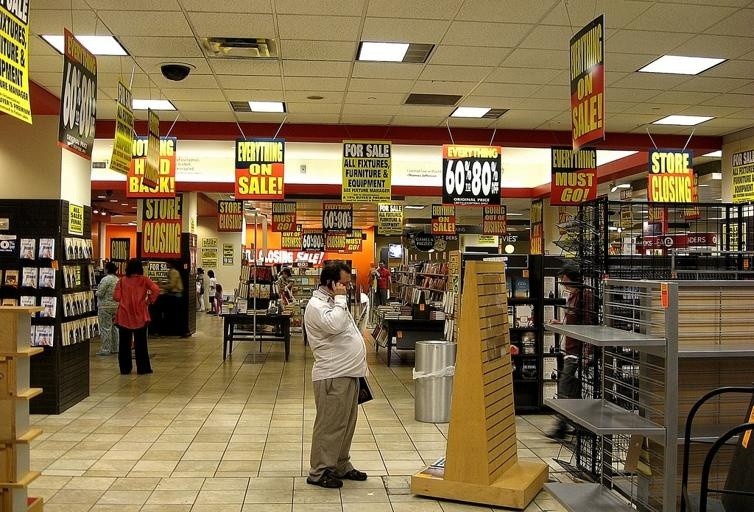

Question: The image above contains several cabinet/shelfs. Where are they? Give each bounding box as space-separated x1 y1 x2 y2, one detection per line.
542 277 754 512
459 252 737 415
0 306 44 512
240 259 352 333
219 313 292 362
371 310 449 367
380 247 402 298
394 271 448 309
553 193 754 483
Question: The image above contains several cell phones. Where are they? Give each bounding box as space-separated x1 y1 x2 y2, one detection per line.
327 279 337 290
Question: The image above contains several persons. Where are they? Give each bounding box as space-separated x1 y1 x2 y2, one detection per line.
94 261 120 356
544 260 598 439
158 259 185 335
214 284 224 316
206 270 218 314
196 268 205 312
113 257 162 375
275 266 295 304
370 259 393 305
303 262 370 490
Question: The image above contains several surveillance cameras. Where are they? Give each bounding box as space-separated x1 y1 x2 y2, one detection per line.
157 62 197 81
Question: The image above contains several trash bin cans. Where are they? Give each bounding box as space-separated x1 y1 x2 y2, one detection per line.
415 340 457 423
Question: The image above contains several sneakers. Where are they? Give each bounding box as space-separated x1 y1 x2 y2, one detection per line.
197 307 223 317
306 472 344 489
336 468 368 481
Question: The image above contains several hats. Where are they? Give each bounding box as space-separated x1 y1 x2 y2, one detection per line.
283 268 292 278
557 264 579 276
